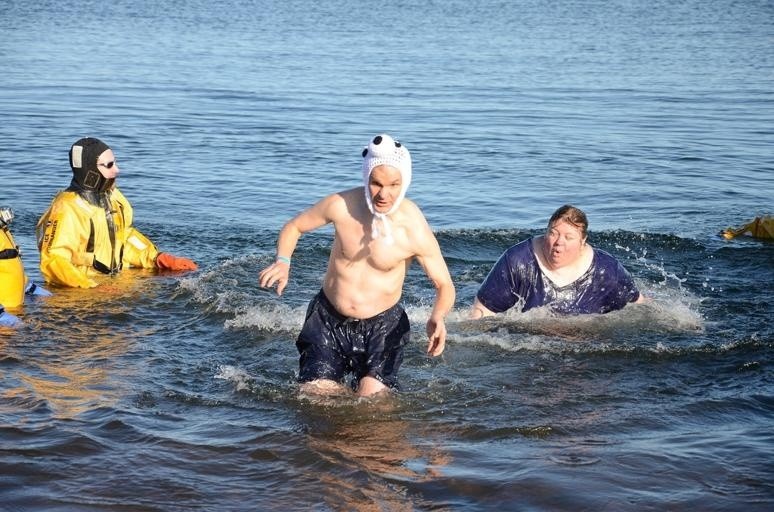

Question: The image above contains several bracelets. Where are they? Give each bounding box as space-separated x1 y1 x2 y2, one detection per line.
276 256 290 264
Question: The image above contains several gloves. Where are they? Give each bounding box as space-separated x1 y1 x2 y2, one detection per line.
157 253 196 271
25 282 52 296
0 303 21 326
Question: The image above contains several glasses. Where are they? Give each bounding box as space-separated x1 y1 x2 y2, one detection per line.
98 161 114 168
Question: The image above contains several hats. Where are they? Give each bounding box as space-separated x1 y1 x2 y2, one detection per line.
361 134 412 243
69 139 116 191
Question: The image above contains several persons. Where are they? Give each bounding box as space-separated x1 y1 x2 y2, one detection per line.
468 205 645 321
37 138 198 289
259 134 455 397
0 207 53 328
